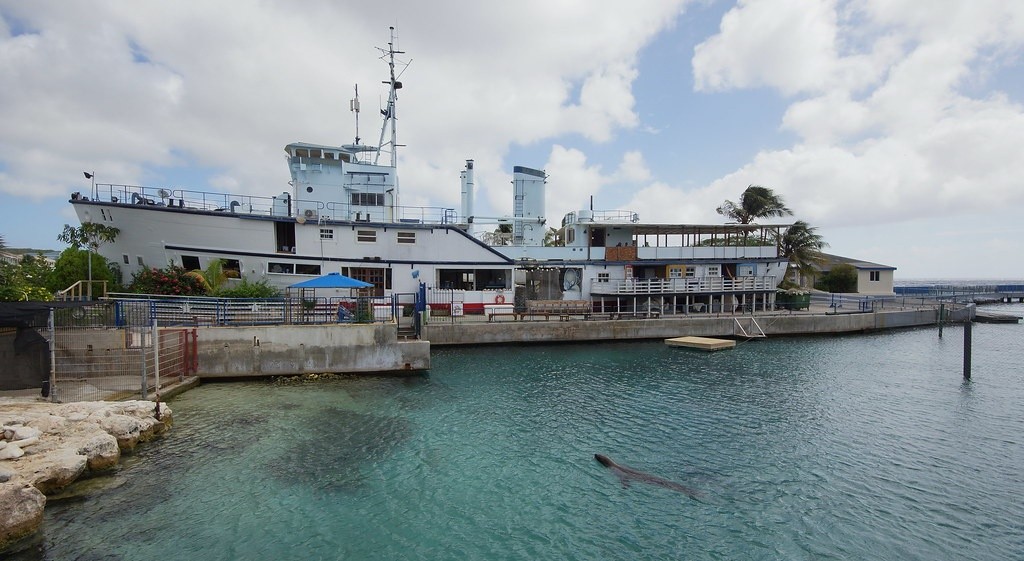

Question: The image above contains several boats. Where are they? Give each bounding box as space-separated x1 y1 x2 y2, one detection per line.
67 22 797 321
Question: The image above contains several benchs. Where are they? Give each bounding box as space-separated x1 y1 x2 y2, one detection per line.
487 312 518 322
549 311 659 324
520 312 550 321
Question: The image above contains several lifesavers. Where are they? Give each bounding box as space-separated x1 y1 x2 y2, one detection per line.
495 295 505 305
